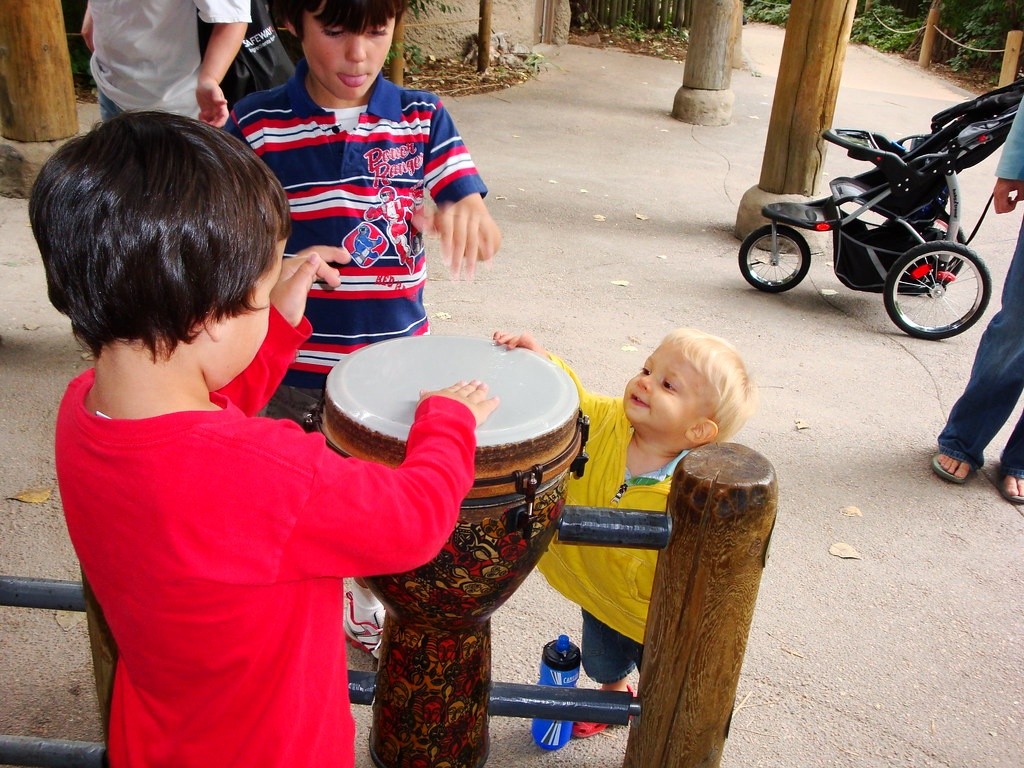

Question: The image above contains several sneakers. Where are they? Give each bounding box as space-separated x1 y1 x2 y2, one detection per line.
346 590 385 659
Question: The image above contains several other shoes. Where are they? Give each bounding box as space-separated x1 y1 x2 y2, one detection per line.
572 682 636 739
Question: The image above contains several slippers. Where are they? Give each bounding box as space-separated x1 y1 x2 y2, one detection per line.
932 452 969 483
997 449 1024 502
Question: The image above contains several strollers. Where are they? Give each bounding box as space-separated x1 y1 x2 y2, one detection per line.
737 70 1024 341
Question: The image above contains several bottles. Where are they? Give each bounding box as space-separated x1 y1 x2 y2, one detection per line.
531 635 581 750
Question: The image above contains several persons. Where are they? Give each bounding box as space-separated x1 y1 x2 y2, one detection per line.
80 0 251 128
493 329 758 738
27 110 502 768
932 95 1024 503
222 0 501 664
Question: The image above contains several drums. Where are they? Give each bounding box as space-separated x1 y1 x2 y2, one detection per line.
321 334 585 768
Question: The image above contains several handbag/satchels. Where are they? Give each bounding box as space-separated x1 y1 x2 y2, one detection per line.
195 0 297 110
